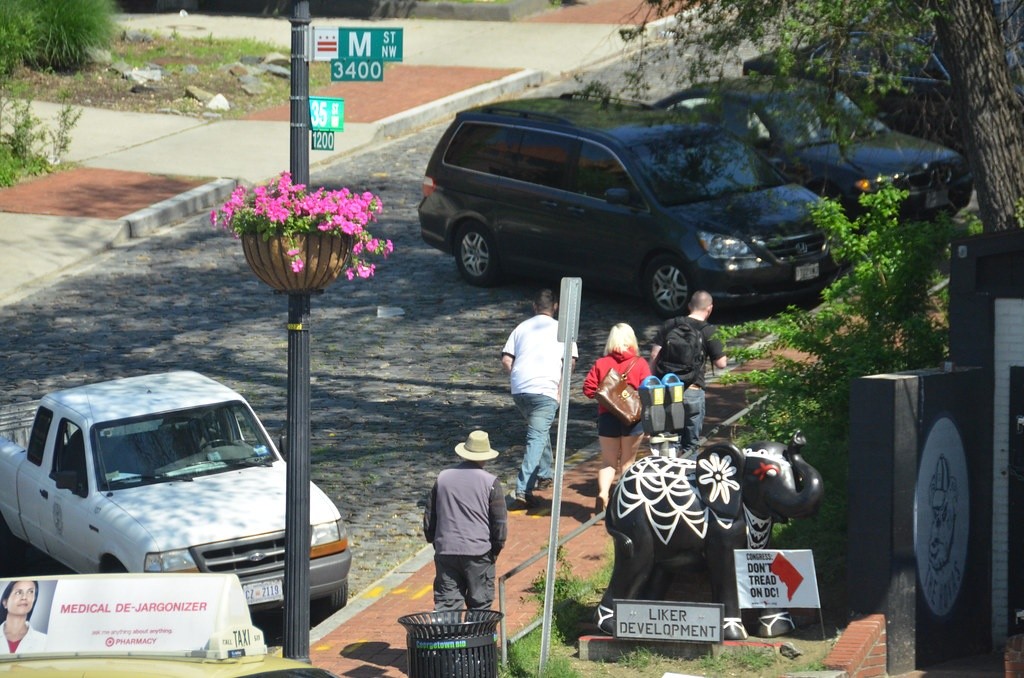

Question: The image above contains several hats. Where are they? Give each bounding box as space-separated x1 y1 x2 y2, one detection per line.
454 430 498 461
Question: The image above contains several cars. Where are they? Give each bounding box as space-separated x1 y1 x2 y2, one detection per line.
738 24 967 152
648 73 972 234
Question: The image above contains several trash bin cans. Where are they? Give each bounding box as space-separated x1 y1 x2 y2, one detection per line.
397 608 505 678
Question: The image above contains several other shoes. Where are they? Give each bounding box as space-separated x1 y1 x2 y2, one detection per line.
596 497 606 520
537 478 551 490
516 493 533 503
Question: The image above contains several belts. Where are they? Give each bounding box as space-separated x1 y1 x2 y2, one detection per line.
688 384 702 390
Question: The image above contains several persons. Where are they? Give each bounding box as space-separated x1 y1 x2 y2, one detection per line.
0 581 46 655
649 291 727 459
583 323 652 520
501 290 579 506
422 430 507 623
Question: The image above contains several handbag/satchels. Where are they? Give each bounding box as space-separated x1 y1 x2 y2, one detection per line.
595 356 643 425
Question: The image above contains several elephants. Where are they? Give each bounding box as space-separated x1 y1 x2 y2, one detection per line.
592 429 826 641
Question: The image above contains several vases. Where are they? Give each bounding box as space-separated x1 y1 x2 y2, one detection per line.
242 223 357 291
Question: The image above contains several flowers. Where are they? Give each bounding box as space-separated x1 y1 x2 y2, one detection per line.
210 173 395 281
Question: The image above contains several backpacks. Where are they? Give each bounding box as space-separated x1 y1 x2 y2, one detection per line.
654 316 709 389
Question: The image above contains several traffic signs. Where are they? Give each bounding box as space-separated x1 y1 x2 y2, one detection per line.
310 96 347 135
307 28 404 82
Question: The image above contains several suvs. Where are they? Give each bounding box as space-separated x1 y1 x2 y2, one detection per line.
417 89 853 319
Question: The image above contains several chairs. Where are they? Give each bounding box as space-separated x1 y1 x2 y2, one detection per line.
67 429 101 474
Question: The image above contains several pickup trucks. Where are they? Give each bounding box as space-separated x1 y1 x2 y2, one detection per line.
1 369 354 635
